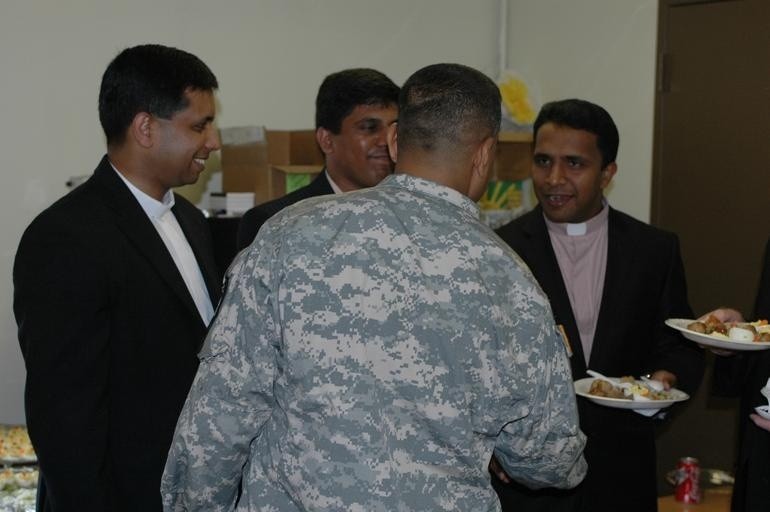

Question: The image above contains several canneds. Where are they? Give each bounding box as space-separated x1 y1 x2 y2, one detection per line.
675 457 701 505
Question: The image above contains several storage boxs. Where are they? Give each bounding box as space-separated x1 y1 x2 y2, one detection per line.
217 124 326 206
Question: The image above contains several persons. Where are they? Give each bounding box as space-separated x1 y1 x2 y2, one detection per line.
691 243 770 512
237 68 400 252
487 97 706 512
158 62 589 512
13 44 223 512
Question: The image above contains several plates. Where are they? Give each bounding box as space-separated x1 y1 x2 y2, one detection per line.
573 376 690 412
665 318 769 351
